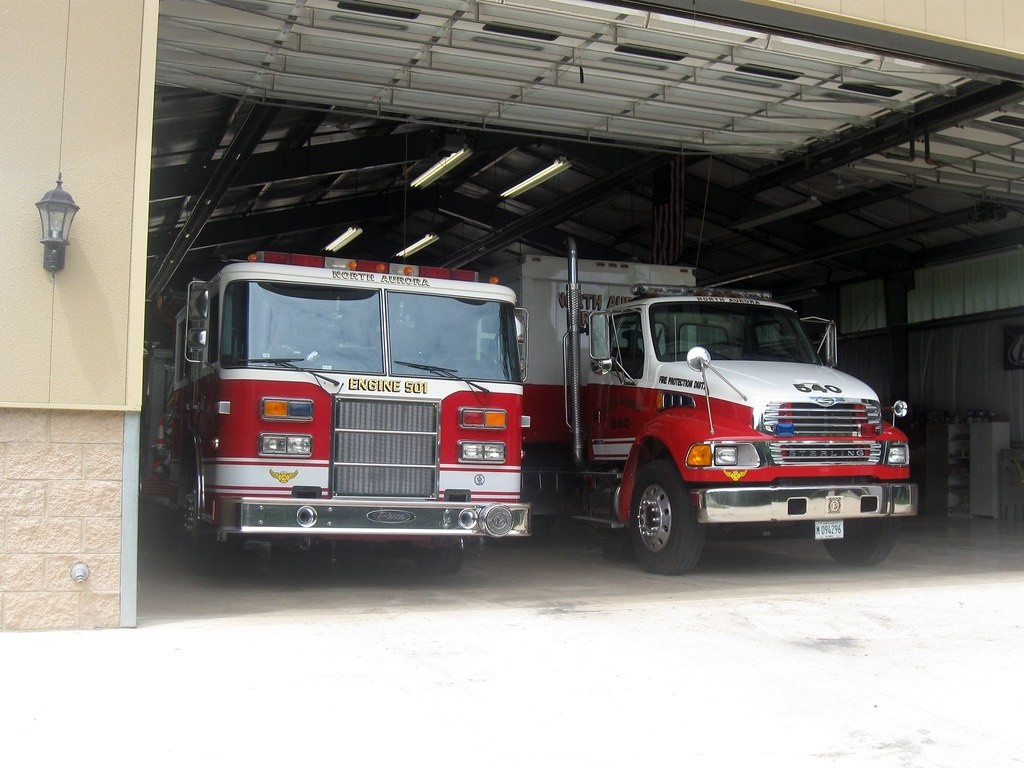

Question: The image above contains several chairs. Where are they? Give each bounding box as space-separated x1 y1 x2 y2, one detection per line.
1001 450 1024 522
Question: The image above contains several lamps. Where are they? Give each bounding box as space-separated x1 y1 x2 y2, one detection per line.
34 171 80 277
391 229 438 259
324 223 362 251
406 132 472 190
492 138 576 200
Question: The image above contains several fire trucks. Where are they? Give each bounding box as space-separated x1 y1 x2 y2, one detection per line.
474 236 920 576
138 250 531 578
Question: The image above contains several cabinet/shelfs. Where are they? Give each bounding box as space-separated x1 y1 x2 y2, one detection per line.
926 421 1010 522
